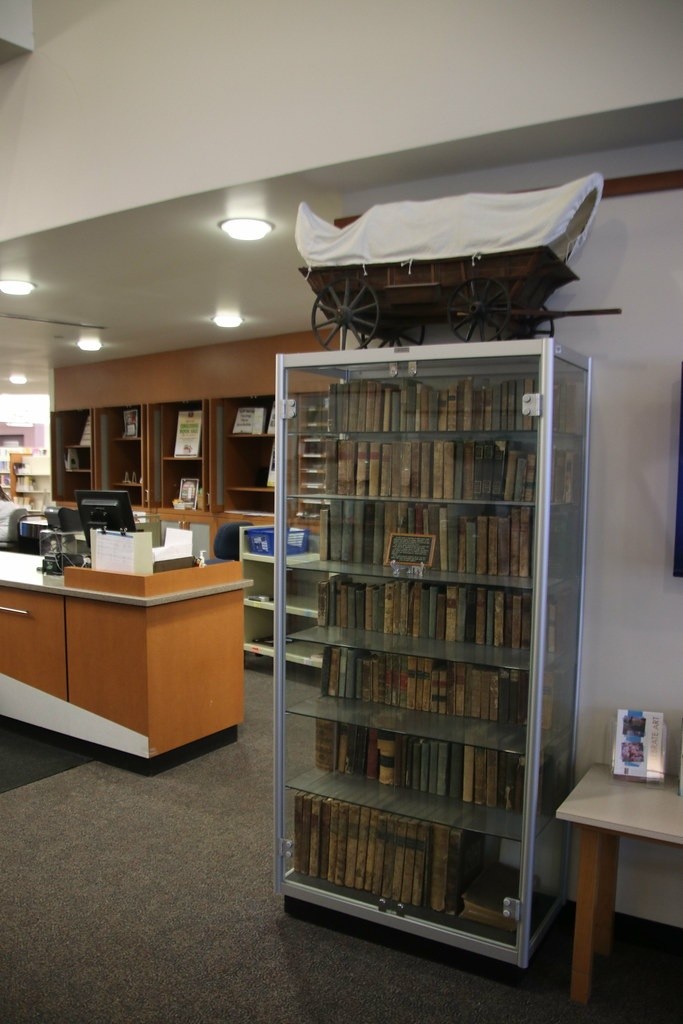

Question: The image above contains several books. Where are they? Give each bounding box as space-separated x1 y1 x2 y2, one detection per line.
290 382 570 916
0 449 53 512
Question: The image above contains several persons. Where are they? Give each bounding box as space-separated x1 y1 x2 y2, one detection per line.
0 487 30 542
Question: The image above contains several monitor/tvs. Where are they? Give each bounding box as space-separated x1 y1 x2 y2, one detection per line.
73 489 137 548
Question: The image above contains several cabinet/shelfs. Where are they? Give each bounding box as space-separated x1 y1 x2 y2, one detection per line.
9 452 52 508
277 339 592 985
0 548 254 776
53 326 346 561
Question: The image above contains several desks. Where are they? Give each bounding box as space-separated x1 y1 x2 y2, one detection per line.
557 763 682 1005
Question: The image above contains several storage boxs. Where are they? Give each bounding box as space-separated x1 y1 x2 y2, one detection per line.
248 528 309 556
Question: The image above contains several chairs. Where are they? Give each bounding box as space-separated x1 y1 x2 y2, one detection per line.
207 522 252 565
